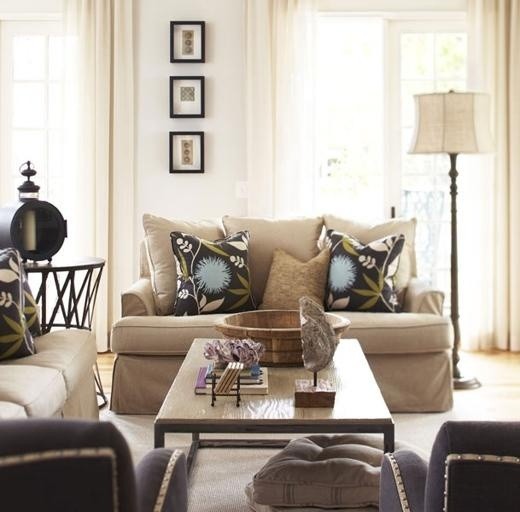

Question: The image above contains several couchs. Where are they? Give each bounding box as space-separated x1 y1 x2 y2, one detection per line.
0 326 100 424
108 233 454 415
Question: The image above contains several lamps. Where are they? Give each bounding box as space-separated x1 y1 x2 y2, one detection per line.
408 87 495 392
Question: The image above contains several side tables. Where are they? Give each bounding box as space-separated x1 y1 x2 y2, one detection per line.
32 254 108 410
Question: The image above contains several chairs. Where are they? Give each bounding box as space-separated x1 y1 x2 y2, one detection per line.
0 421 188 512
379 419 520 512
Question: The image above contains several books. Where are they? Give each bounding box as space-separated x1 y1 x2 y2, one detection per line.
193 366 270 394
203 363 261 384
214 362 244 394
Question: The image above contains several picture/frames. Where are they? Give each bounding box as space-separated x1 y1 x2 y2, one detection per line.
169 21 205 173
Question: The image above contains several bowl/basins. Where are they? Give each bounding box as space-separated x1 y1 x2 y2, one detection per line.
212 308 353 368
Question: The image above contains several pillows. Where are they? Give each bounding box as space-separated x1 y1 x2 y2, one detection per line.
139 213 421 314
0 245 42 361
